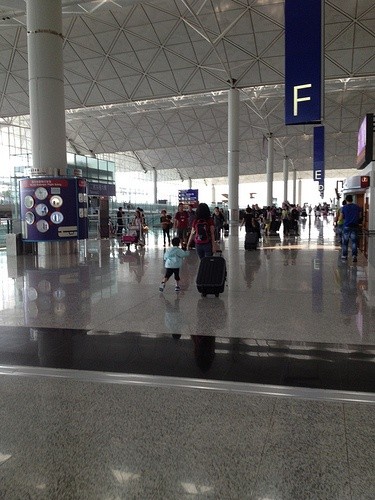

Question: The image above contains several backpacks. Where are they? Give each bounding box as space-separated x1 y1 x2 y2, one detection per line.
195 217 212 244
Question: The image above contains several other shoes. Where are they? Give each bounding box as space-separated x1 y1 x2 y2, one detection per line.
159 285 164 292
175 287 180 291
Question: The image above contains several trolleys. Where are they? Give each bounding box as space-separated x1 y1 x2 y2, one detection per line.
263 220 281 241
283 218 301 237
124 220 145 250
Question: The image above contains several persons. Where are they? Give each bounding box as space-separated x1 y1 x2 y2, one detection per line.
334 258 360 325
212 207 226 244
260 235 300 265
189 323 216 373
133 207 145 235
117 247 124 264
159 293 191 340
186 203 219 260
241 264 254 289
130 250 146 283
158 238 190 291
116 207 124 237
341 194 361 263
238 201 329 236
160 211 171 247
173 203 196 251
335 200 346 246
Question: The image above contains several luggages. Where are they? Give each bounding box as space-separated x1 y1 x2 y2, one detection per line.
244 227 258 248
196 250 227 297
121 236 139 242
